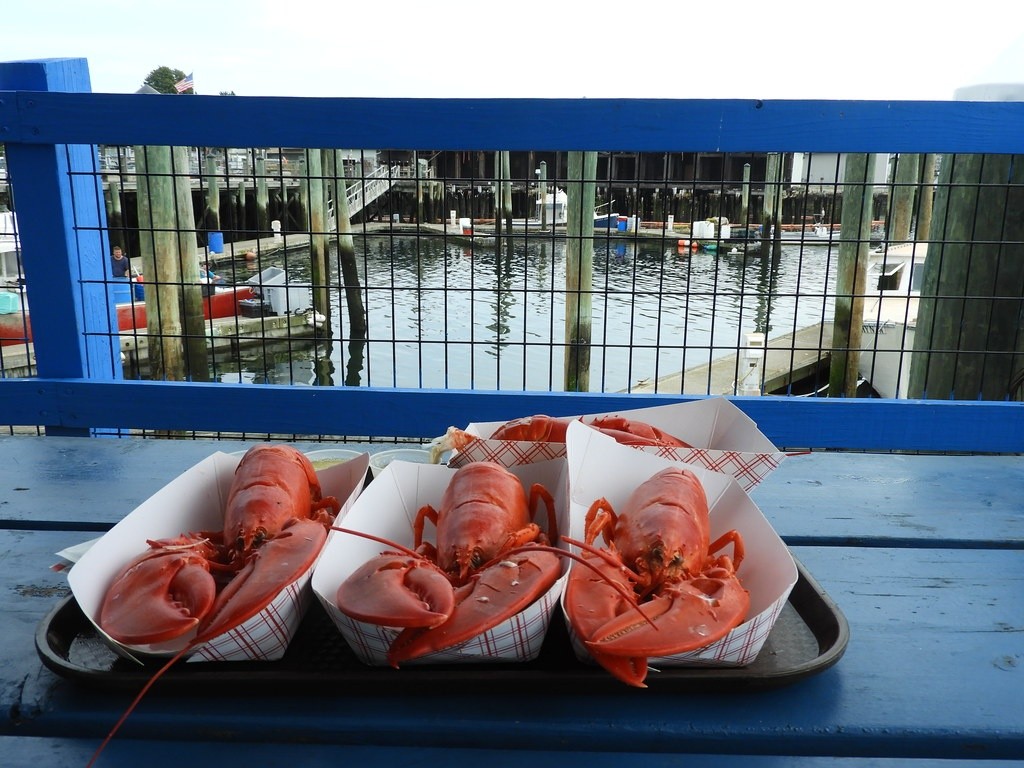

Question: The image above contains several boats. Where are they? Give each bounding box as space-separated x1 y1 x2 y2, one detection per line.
697 241 761 252
771 231 841 245
1 284 316 380
502 186 619 227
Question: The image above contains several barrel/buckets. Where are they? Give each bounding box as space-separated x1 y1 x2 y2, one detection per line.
460 218 470 234
208 232 223 254
617 221 627 231
693 220 730 239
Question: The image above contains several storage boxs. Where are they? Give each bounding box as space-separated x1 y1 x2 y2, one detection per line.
240 300 271 318
248 266 285 294
203 283 215 297
560 417 799 673
309 458 572 668
263 282 312 316
136 285 144 301
449 393 786 508
50 450 372 663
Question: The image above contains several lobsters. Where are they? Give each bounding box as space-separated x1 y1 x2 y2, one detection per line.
560 467 750 689
431 414 695 464
323 462 659 670
82 443 340 768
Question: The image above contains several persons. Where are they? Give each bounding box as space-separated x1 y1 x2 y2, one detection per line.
111 246 129 277
